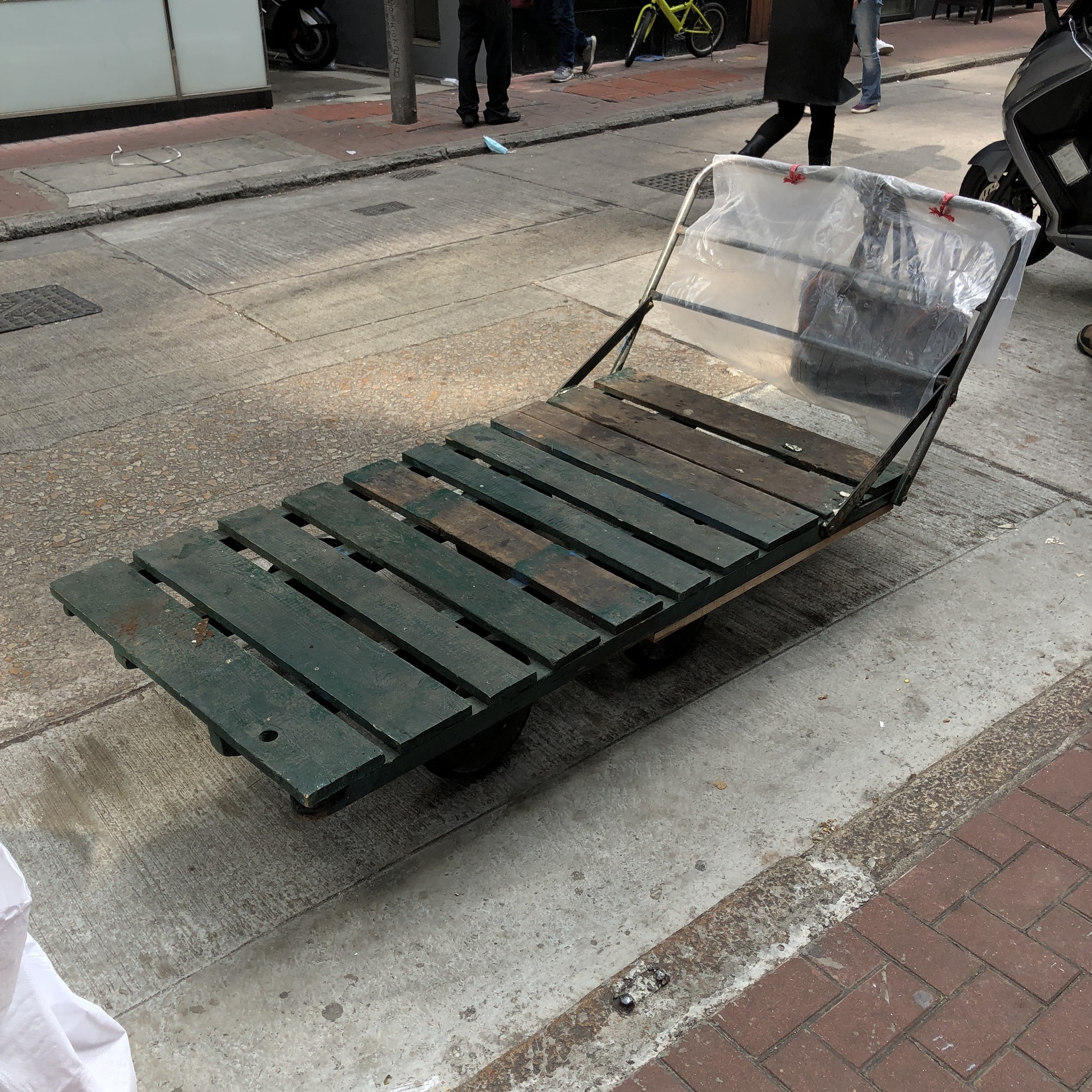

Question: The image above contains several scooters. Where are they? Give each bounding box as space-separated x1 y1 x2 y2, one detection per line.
959 0 1092 264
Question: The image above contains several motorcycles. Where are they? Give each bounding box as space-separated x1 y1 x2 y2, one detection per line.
258 0 338 71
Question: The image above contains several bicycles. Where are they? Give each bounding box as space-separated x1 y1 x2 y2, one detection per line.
626 0 729 67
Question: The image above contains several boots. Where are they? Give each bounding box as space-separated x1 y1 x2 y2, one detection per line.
737 133 771 159
808 149 831 166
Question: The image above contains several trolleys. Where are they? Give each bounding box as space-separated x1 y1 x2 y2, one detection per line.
43 147 1044 817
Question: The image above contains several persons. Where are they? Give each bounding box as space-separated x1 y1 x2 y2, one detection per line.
853 33 895 55
454 0 522 128
535 0 597 83
723 0 860 168
794 0 881 116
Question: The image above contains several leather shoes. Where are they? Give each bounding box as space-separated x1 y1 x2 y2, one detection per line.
485 111 522 124
463 112 478 124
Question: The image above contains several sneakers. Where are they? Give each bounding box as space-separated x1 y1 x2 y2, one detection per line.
851 102 881 114
877 40 895 55
549 65 575 82
581 36 596 74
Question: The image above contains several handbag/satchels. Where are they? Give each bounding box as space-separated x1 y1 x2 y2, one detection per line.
790 178 965 417
